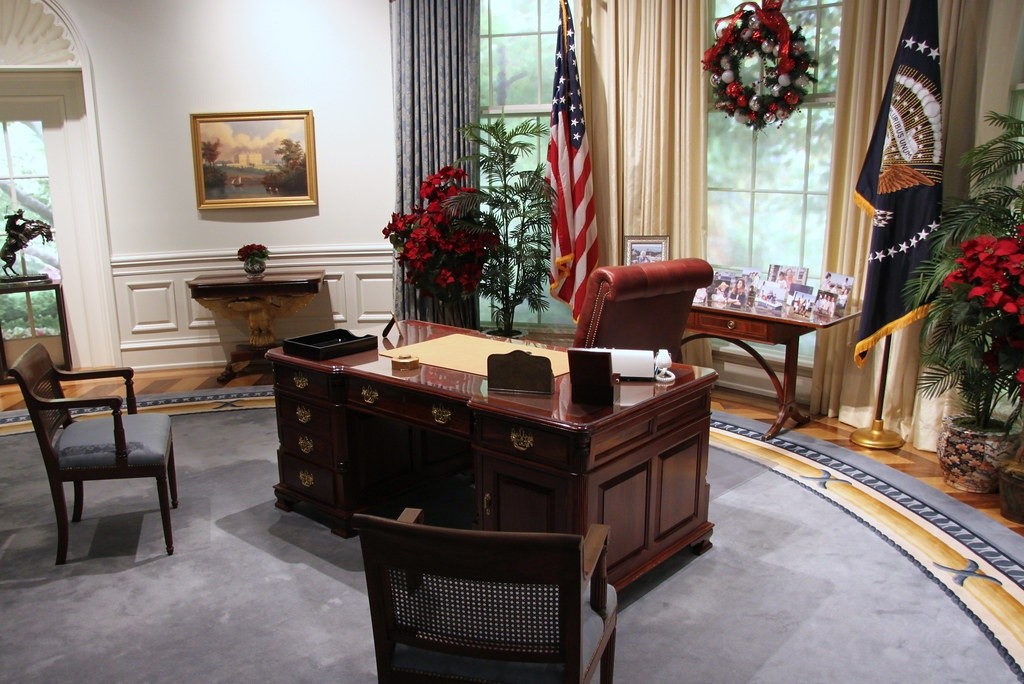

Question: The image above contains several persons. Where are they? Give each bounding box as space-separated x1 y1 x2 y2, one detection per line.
638 249 847 320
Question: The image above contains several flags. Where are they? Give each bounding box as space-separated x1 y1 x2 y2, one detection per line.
542 0 599 324
849 1 945 369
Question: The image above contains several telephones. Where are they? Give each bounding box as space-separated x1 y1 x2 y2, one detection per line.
653 348 672 371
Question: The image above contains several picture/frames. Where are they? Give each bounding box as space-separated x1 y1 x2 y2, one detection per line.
623 234 670 267
190 111 318 211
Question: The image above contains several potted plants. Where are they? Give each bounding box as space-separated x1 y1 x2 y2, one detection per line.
902 113 1024 495
440 105 558 340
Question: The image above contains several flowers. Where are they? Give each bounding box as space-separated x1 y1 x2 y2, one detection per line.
943 224 1024 405
382 165 501 330
237 244 270 261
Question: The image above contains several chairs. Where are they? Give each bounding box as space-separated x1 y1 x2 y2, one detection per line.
571 258 714 364
7 343 179 565
353 507 618 684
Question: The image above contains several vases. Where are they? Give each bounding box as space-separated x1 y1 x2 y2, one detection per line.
998 465 1024 522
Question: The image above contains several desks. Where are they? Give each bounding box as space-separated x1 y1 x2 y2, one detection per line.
190 272 326 385
244 257 266 278
680 302 863 441
0 279 73 383
266 321 718 596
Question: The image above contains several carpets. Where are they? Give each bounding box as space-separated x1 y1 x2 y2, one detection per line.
0 388 1024 684
